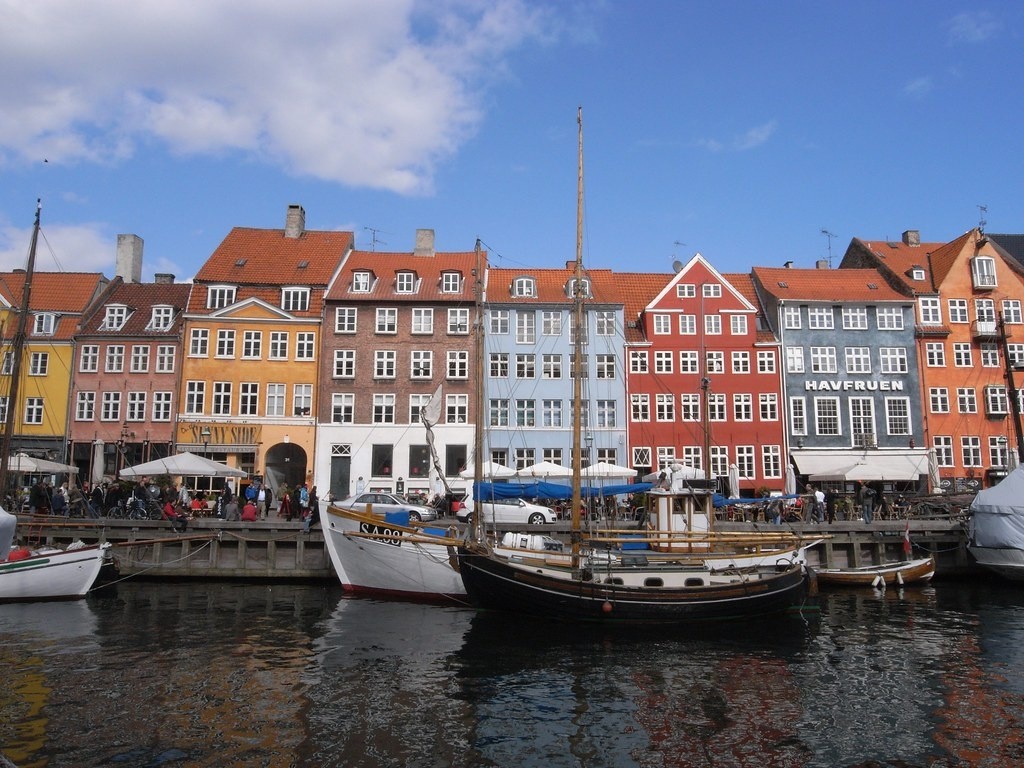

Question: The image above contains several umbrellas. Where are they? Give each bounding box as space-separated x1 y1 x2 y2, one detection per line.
459 460 716 482
0 453 79 473
729 463 740 500
784 464 797 507
120 451 247 478
810 460 919 480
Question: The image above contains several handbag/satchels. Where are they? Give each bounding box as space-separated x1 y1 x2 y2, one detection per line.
865 489 877 497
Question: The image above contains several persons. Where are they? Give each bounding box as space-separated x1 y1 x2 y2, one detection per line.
402 493 447 519
727 479 911 526
30 471 320 532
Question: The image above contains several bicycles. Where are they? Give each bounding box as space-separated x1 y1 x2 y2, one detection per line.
106 499 163 520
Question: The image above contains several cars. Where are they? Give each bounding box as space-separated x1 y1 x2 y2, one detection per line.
455 489 557 527
324 490 437 523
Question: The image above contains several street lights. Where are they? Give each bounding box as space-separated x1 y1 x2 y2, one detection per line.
200 426 212 518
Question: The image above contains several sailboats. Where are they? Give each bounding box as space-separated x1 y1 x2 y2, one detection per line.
317 236 626 607
449 102 836 634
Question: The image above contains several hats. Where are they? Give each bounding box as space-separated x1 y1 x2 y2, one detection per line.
247 501 253 503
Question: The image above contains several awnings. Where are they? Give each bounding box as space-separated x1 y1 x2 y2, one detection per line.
790 450 933 475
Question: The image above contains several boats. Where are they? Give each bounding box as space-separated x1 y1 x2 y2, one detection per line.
0 505 113 603
809 556 936 587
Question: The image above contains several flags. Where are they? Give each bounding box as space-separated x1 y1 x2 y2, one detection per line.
903 521 910 555
